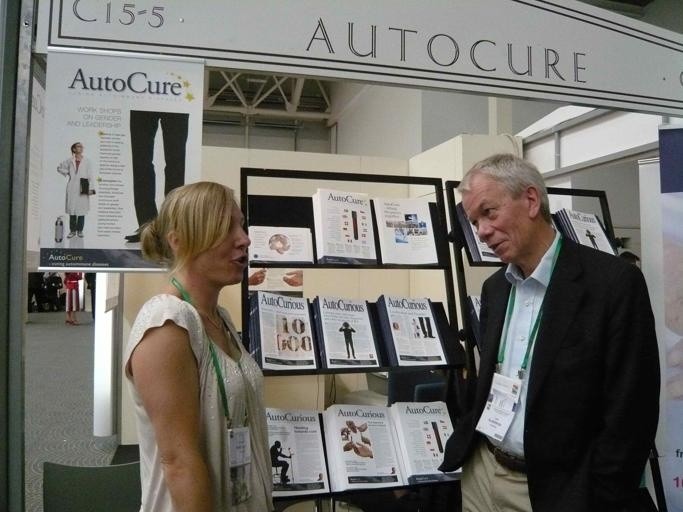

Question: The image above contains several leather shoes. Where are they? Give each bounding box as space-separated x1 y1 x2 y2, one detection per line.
126 229 143 242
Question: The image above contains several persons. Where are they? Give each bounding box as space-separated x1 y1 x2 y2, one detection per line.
121 112 190 243
83 272 95 320
55 140 92 240
62 271 85 328
122 177 276 512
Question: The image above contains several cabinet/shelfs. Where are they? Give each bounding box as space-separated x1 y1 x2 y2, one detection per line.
238 166 461 510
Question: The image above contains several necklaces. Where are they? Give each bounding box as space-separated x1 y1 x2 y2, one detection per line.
191 302 226 337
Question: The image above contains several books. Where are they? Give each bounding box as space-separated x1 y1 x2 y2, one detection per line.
458 199 616 268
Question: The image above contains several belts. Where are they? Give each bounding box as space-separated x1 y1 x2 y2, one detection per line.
481 433 526 474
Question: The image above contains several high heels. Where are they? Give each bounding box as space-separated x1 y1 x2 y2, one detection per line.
64 319 79 325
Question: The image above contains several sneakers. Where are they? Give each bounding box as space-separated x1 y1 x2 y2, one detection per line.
67 231 84 238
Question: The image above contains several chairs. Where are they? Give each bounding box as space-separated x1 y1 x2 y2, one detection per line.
36 459 142 511
